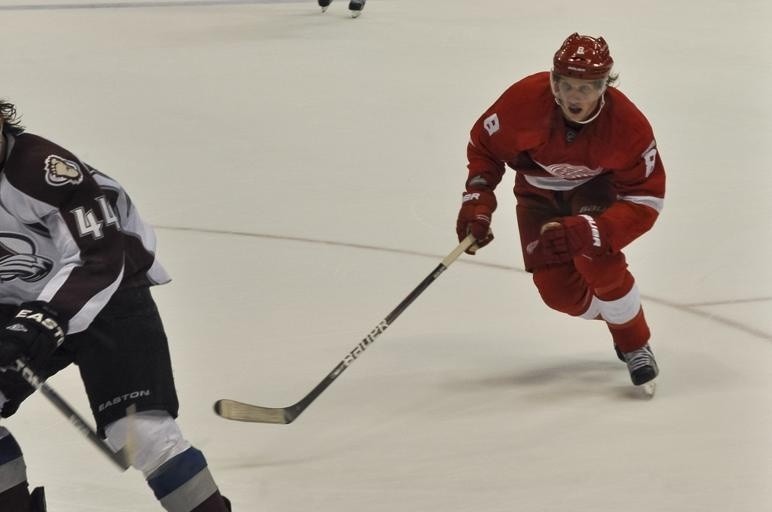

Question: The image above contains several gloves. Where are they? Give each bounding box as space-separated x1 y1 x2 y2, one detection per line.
540 213 606 263
0 300 69 401
457 188 497 255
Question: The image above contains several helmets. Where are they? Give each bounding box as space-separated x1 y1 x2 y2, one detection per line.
550 33 613 103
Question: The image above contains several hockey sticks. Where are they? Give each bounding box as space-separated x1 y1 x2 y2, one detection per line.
214 230 477 424
13 362 138 470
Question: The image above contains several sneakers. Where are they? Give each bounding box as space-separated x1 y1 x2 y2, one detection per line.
622 342 658 385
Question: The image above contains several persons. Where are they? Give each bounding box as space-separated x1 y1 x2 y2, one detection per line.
317 1 369 12
452 29 666 389
0 99 239 510
